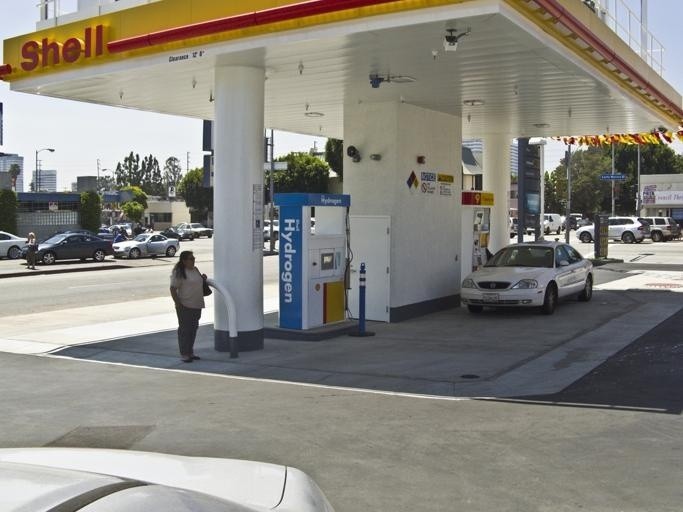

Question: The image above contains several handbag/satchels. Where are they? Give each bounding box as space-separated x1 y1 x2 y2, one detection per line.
33 244 38 251
201 274 212 296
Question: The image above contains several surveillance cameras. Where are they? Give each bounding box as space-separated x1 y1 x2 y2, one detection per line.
442 35 458 52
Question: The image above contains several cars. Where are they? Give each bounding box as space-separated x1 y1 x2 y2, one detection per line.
160 222 213 241
263 218 279 243
111 232 181 259
33 231 114 265
458 239 595 316
0 230 31 259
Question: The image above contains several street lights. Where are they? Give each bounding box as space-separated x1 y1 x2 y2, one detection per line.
102 168 115 180
35 148 56 192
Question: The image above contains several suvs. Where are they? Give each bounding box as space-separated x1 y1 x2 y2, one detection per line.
574 215 681 245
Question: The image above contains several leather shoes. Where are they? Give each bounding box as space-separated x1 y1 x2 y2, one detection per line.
181 355 200 362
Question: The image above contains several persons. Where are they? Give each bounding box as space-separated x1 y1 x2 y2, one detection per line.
26 232 38 271
112 223 154 242
169 249 208 363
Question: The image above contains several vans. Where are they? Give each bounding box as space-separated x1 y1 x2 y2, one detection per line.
525 213 562 235
